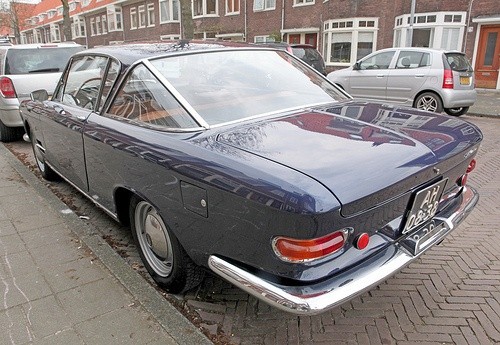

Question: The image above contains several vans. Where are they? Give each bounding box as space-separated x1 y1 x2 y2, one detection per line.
1 42 86 143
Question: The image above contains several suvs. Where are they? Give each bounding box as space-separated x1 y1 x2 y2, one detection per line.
248 42 327 76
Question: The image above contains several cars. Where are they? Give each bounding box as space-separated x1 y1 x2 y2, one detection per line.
18 41 484 317
326 47 476 117
